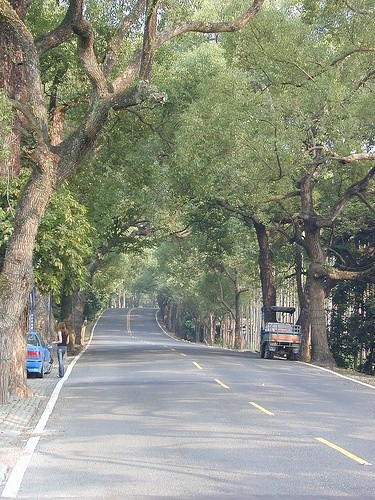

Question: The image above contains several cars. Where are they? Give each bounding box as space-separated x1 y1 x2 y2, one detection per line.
26 331 53 378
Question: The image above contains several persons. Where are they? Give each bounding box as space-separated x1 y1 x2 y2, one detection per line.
51 321 69 377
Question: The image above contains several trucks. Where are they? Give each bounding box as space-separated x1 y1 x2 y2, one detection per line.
259 304 301 360
